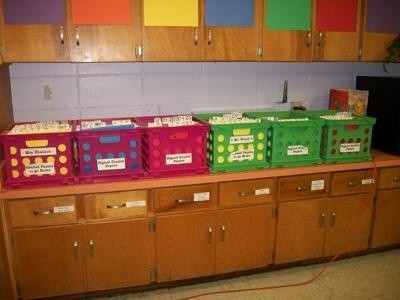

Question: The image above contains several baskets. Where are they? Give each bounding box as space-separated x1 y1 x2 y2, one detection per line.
1 109 377 188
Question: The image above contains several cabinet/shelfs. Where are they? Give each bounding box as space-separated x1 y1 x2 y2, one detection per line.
372 150 400 253
155 174 278 287
1 0 143 64
4 184 157 297
141 0 262 61
276 162 378 266
360 0 400 64
260 0 363 63
